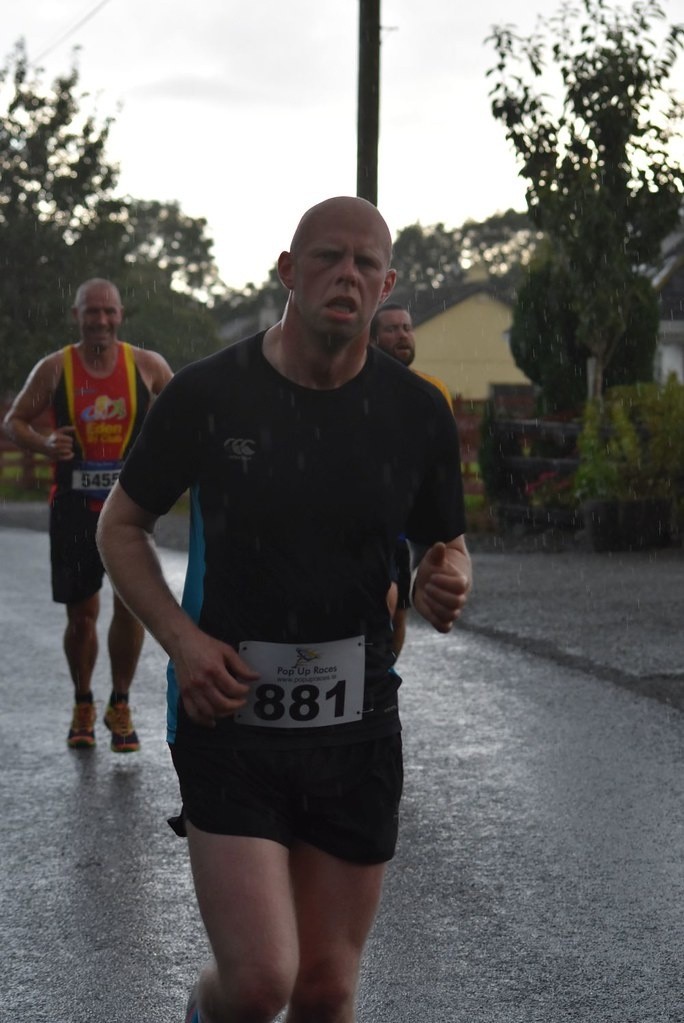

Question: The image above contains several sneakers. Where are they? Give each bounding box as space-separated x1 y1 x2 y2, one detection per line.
103 702 140 752
67 703 97 748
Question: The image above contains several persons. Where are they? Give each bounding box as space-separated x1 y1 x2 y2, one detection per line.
0 196 472 1022
